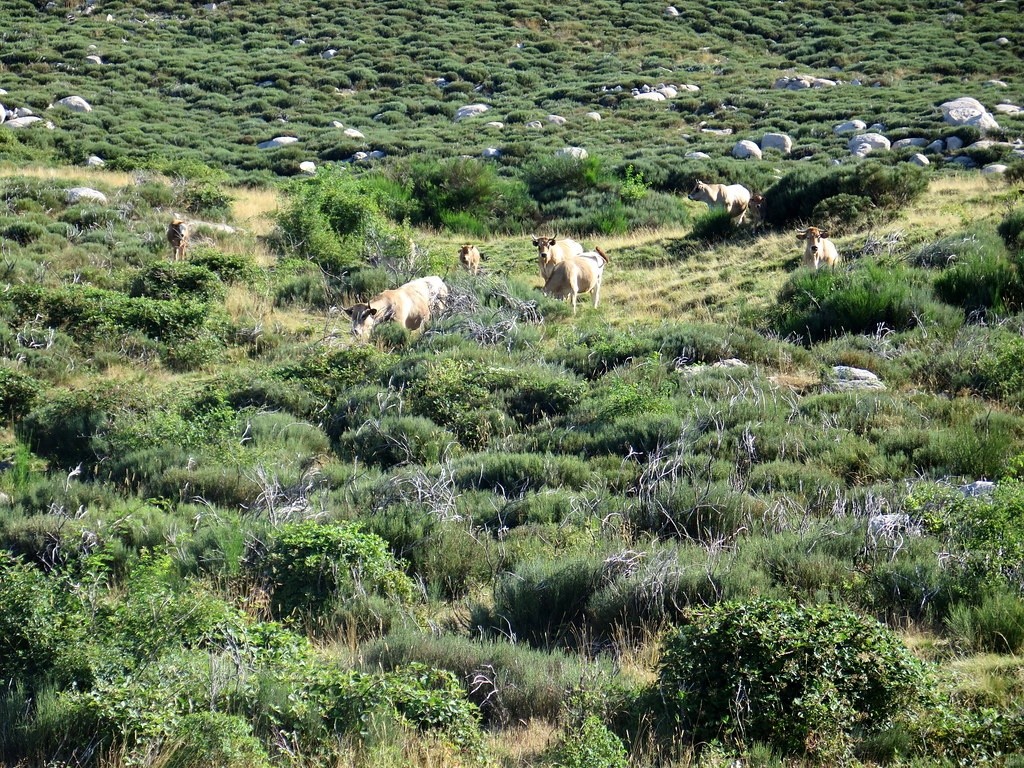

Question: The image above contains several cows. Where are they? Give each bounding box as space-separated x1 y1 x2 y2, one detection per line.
688 177 751 231
796 226 839 272
167 219 189 261
340 275 449 354
456 243 481 279
529 232 607 315
